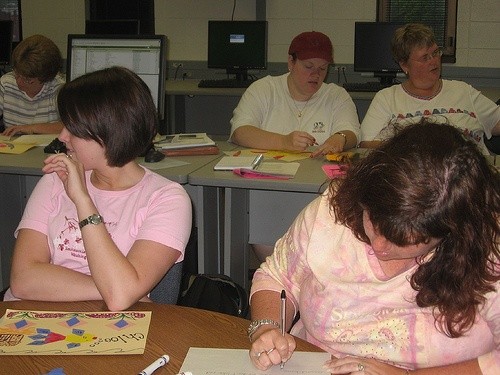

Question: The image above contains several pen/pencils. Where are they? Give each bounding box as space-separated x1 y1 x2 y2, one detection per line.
280 290 286 369
315 142 319 145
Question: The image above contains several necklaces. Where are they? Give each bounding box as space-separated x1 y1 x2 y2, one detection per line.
401 80 442 100
291 93 312 117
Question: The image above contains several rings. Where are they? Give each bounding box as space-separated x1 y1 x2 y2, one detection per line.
255 351 266 361
359 363 365 372
266 348 276 355
66 154 72 158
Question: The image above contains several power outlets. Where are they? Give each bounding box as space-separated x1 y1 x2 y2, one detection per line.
178 63 184 68
341 66 347 71
172 63 178 68
335 66 341 71
184 72 193 78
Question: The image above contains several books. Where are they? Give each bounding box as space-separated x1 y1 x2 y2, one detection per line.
214 154 301 179
153 131 220 156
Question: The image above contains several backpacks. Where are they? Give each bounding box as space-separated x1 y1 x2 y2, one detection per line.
177 272 247 321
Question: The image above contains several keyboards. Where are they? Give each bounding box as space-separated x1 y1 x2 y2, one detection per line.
342 82 400 92
44 137 70 156
198 79 256 89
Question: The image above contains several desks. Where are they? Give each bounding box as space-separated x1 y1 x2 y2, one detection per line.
0 74 500 375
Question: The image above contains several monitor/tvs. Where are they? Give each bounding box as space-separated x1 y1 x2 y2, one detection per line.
64 32 169 122
353 21 405 81
206 19 268 78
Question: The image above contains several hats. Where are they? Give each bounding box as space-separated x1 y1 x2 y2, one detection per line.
288 31 334 62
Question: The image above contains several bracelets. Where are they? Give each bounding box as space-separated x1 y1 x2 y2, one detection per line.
335 132 347 145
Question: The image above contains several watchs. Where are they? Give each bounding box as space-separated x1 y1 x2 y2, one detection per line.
79 213 104 229
249 319 280 343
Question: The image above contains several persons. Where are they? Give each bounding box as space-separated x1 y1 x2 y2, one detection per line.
248 115 500 375
0 66 192 312
360 23 500 154
0 35 65 136
228 31 361 158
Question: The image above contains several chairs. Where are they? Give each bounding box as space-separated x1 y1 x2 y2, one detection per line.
149 261 182 305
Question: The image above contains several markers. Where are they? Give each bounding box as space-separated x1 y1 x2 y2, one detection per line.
137 355 170 375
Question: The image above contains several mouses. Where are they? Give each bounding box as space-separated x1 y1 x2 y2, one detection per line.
144 151 165 163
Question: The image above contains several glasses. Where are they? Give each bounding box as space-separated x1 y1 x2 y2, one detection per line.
12 68 39 85
409 47 443 63
